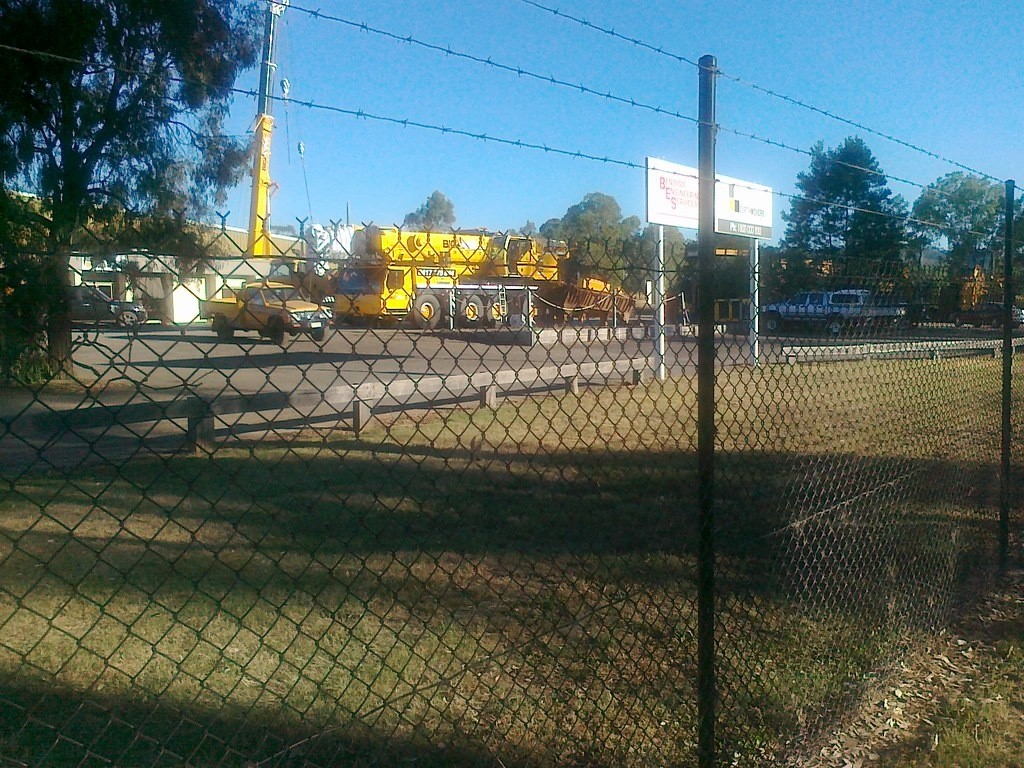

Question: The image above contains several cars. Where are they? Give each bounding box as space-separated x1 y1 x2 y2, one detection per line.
897 299 1023 333
64 283 147 327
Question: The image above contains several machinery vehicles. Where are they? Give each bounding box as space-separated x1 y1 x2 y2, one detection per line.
323 224 636 329
242 0 335 335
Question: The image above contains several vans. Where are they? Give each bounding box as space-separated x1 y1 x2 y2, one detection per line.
828 288 874 305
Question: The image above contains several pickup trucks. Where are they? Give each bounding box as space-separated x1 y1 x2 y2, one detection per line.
759 290 906 339
199 280 333 345
